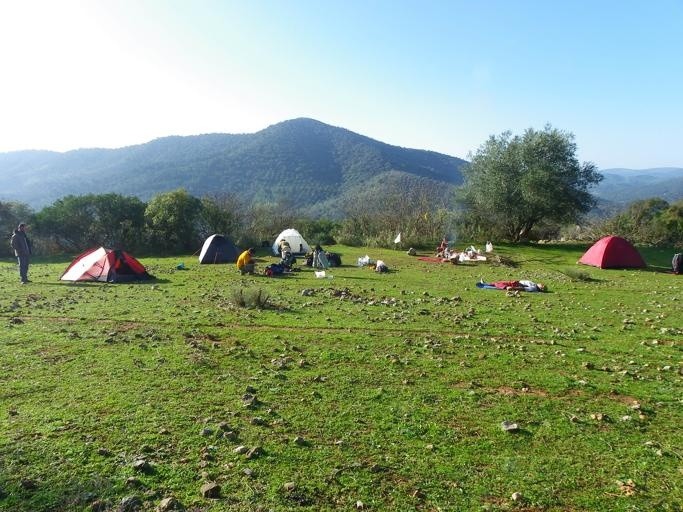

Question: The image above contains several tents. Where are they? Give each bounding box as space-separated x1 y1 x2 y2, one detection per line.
578 236 647 270
271 228 313 257
199 233 238 264
58 246 153 285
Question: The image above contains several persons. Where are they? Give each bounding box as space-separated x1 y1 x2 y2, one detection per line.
441 237 448 250
10 222 31 284
237 248 257 275
481 278 547 291
278 238 291 261
